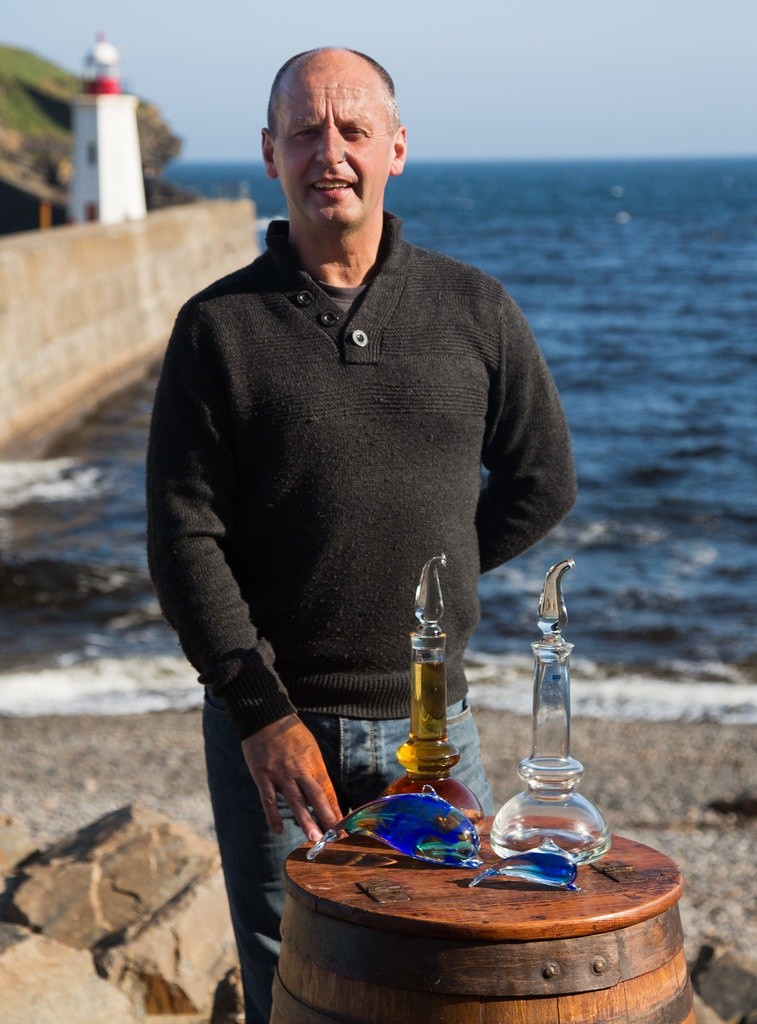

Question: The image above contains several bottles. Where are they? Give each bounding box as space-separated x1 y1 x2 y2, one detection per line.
378 553 487 836
490 556 611 867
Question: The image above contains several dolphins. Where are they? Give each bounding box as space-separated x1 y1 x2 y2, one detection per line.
305 784 582 895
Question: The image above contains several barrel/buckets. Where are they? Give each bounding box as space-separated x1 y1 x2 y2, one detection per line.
268 818 698 1024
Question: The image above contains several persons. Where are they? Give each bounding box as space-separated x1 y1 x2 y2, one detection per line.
145 46 576 1024
